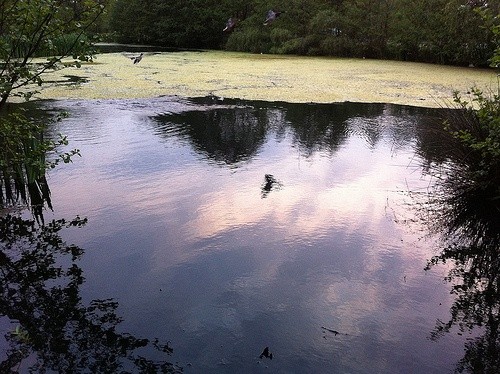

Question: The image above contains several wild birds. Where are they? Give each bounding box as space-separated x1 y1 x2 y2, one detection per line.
130 52 144 65
263 9 288 26
222 16 240 32
